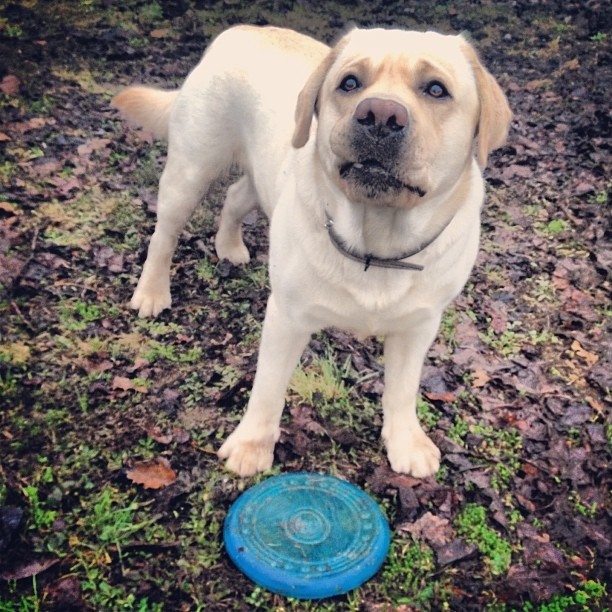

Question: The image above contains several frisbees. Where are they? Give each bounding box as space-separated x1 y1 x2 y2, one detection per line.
222 472 391 598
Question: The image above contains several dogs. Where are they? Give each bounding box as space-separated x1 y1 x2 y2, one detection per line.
109 20 515 480
